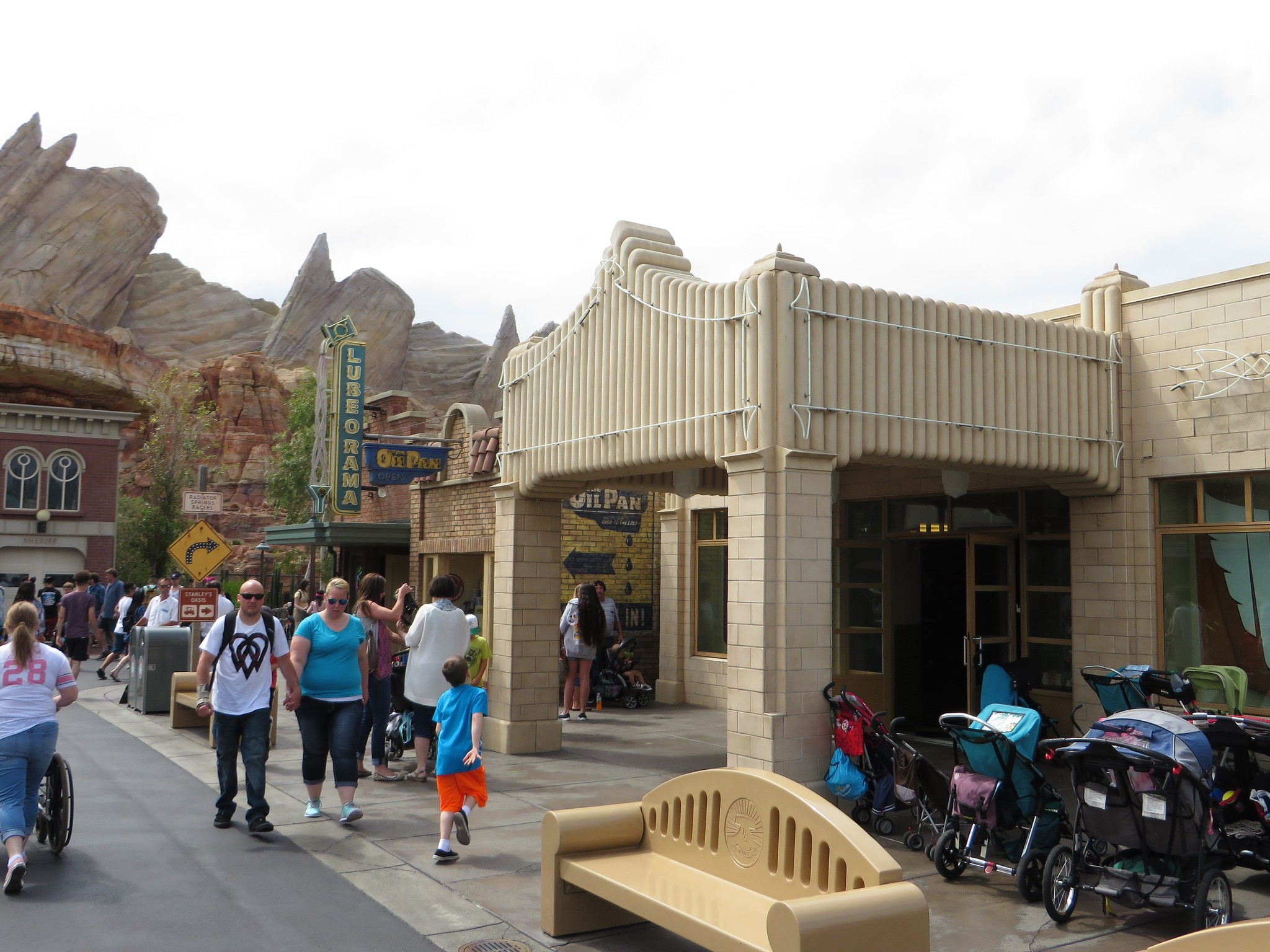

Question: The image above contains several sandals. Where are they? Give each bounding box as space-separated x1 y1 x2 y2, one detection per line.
406 769 427 782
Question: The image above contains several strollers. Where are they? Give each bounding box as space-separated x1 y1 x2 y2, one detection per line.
820 662 1270 935
586 634 650 710
376 647 443 768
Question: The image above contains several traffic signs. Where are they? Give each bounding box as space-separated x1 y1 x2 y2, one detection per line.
178 588 218 624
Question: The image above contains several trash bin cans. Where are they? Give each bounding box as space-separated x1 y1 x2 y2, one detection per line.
134 626 192 715
128 626 142 709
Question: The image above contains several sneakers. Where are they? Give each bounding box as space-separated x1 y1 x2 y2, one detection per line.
339 802 363 822
249 818 274 832
3 856 29 892
304 798 321 817
433 849 459 861
632 683 642 689
642 684 652 690
454 809 471 845
214 813 231 827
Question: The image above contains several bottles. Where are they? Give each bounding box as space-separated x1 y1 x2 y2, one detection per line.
1169 670 1184 692
596 693 602 710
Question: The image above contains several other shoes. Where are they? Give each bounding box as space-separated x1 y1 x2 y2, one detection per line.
558 711 570 720
578 713 587 720
358 771 372 777
374 773 403 780
96 651 122 682
570 707 592 711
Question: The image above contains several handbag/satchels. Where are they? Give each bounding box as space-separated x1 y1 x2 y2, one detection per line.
823 714 869 802
352 601 377 673
393 587 417 626
834 713 864 757
98 617 116 630
948 761 998 830
892 748 920 807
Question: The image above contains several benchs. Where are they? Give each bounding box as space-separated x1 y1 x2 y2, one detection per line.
540 767 931 952
169 671 279 749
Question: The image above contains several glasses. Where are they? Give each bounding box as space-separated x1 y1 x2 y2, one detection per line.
308 584 310 586
327 598 349 605
239 593 264 600
159 584 171 588
595 588 604 590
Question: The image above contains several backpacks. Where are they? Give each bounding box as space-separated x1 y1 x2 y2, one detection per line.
288 591 302 615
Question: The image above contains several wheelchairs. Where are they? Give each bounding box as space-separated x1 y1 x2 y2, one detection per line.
35 749 74 853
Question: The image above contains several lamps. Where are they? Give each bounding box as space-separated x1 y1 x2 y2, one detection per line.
672 468 701 499
36 509 51 533
941 469 970 499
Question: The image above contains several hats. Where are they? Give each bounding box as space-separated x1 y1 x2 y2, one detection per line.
171 573 180 580
465 613 479 635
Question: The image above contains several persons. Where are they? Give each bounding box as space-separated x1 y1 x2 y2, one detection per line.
431 655 489 862
570 582 597 711
282 578 372 824
586 579 624 705
557 581 601 720
306 590 327 615
611 643 653 691
0 561 236 685
193 579 302 833
463 612 494 688
293 578 311 633
353 571 407 783
392 573 471 783
0 596 81 897
